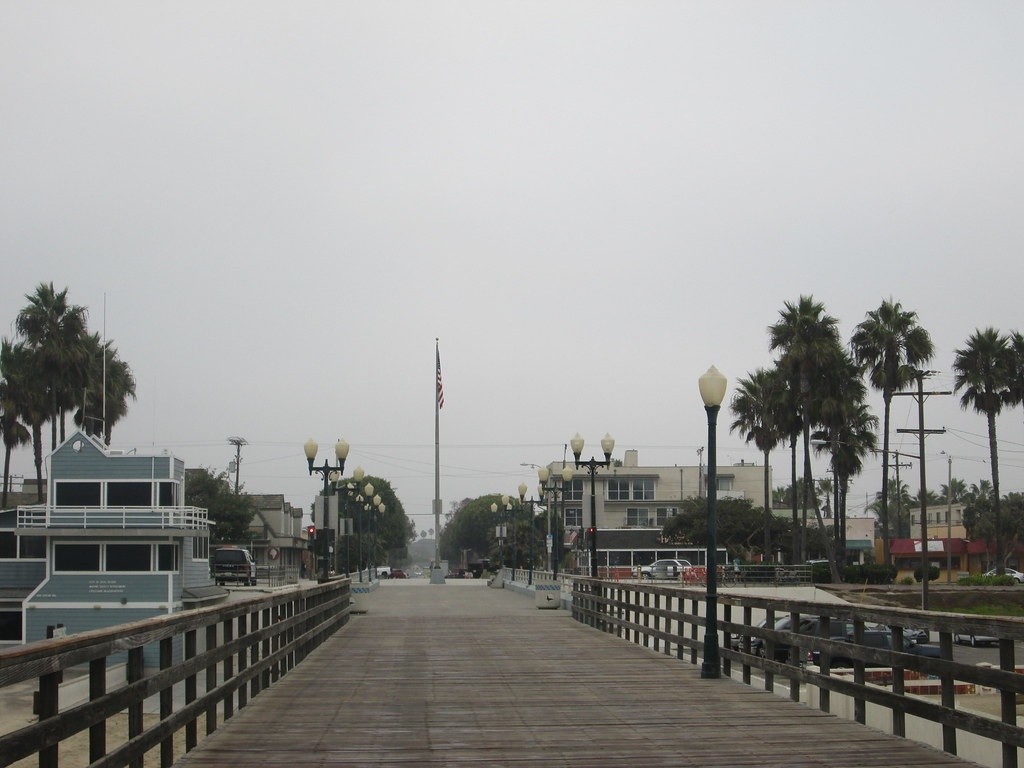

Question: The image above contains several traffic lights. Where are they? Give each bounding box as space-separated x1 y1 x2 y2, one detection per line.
587 527 595 539
307 525 316 551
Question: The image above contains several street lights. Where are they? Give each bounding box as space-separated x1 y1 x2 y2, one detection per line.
362 494 386 582
347 483 374 583
304 435 350 588
537 466 573 581
518 482 546 586
331 465 365 579
693 365 732 672
491 496 514 572
807 438 930 611
569 431 616 608
940 449 953 587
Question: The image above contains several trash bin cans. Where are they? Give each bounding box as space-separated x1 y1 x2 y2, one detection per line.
656 565 667 580
285 568 299 584
350 582 370 614
534 579 561 609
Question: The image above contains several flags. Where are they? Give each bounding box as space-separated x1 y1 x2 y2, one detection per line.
437 355 445 408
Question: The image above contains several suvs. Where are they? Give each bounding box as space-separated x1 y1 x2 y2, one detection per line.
213 546 258 587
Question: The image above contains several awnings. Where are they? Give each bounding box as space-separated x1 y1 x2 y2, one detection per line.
846 540 872 549
889 538 968 554
967 538 1024 554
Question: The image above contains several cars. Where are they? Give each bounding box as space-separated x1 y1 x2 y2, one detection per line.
953 634 1000 646
984 568 1024 585
631 559 691 581
377 566 422 579
740 613 943 669
